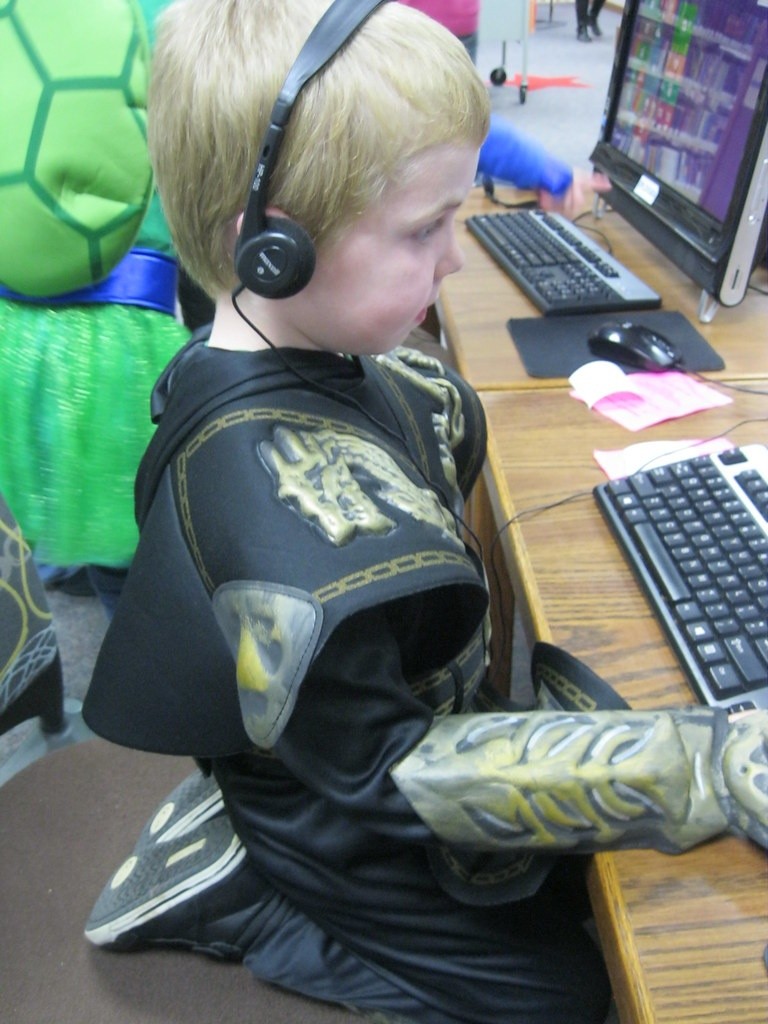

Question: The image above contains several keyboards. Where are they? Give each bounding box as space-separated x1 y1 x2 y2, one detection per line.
465 210 663 317
592 443 768 716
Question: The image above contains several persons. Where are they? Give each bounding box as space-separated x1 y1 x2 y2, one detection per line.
400 1 478 76
82 0 768 1021
4 0 614 750
575 0 605 44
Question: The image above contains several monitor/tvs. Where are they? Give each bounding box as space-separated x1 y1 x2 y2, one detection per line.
588 0 767 306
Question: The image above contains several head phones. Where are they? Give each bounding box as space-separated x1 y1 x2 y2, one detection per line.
232 0 392 300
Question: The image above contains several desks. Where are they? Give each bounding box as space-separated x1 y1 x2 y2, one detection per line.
433 182 768 392
464 379 766 1024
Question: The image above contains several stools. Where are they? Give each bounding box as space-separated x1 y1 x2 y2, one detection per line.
0 737 438 1024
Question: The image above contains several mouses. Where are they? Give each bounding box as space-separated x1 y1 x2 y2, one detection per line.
585 322 683 371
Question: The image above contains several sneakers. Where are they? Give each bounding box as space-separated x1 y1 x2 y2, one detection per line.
84 772 263 964
